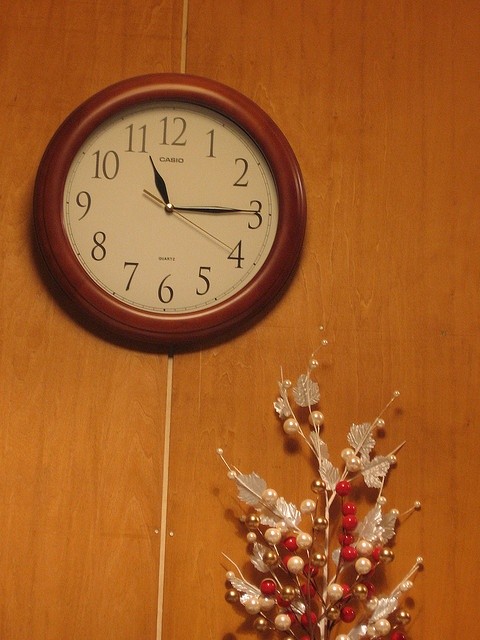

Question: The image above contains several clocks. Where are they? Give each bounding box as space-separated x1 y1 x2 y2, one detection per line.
31 74 306 356
